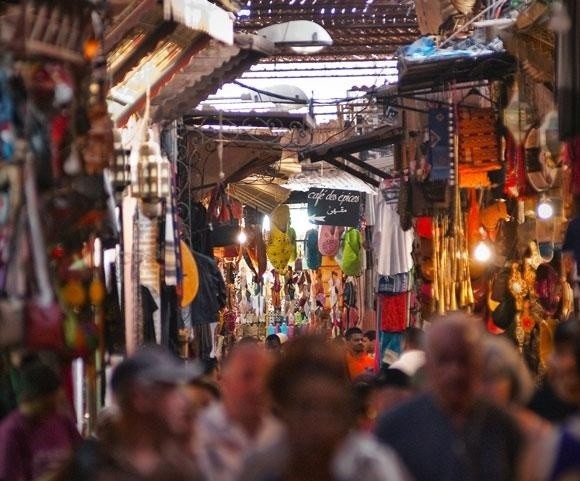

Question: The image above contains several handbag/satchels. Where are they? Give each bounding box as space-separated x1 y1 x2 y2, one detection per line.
210 200 243 258
318 224 340 256
480 198 507 230
503 156 526 197
398 179 447 321
491 265 516 330
1 253 108 353
3 67 121 248
494 216 518 257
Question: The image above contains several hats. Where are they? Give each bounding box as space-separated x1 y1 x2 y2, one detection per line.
10 360 58 400
388 350 428 377
112 349 205 392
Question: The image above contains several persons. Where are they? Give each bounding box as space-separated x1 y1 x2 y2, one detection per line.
1 310 580 481
